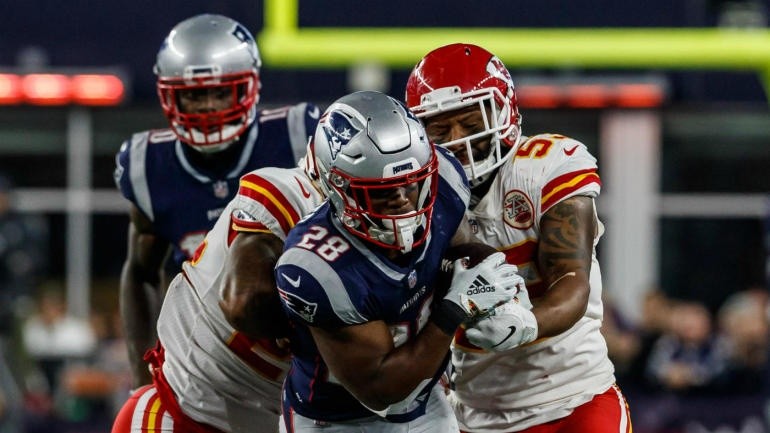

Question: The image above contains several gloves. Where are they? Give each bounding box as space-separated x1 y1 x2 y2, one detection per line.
464 304 539 353
440 251 525 323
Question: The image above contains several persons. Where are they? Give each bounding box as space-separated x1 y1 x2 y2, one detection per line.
109 136 331 433
111 14 324 389
402 41 636 433
0 272 769 433
273 90 526 433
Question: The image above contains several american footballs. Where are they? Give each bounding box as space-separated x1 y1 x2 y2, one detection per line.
442 244 508 303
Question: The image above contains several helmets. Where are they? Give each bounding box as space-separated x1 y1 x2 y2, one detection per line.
155 15 263 152
313 91 436 251
405 43 520 187
